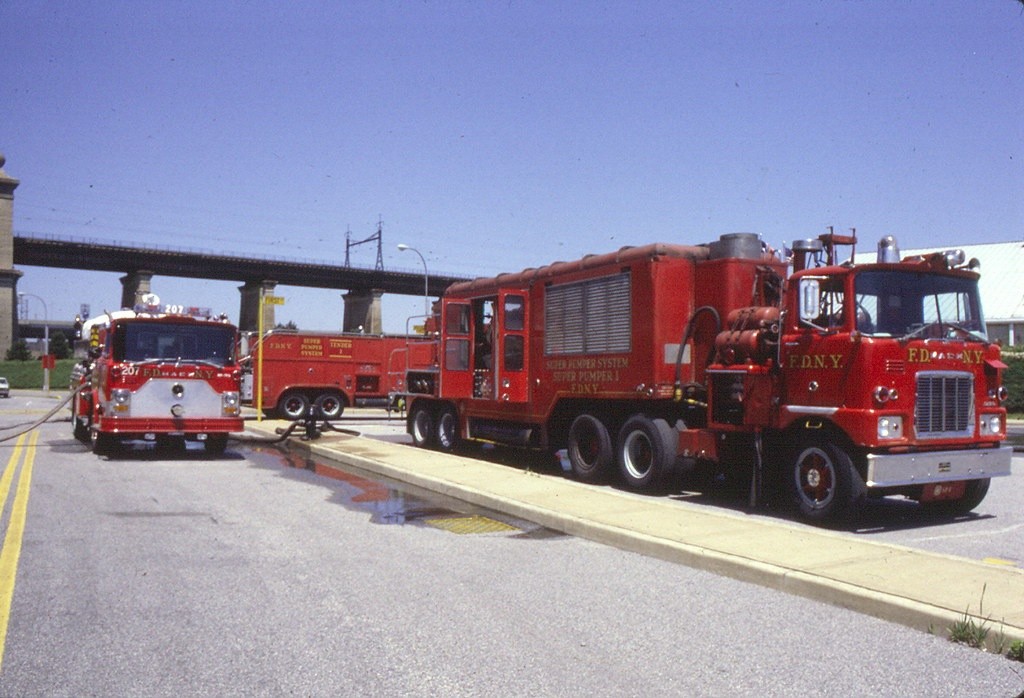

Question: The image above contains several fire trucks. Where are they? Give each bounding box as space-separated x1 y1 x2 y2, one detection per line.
68 294 245 455
386 226 1012 531
237 328 439 421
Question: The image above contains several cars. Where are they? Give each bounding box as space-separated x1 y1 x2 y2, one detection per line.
0 377 9 398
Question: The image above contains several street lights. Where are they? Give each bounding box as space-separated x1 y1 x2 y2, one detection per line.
19 292 50 390
397 245 428 313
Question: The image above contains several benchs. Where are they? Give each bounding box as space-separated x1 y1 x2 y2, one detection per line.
814 313 873 333
